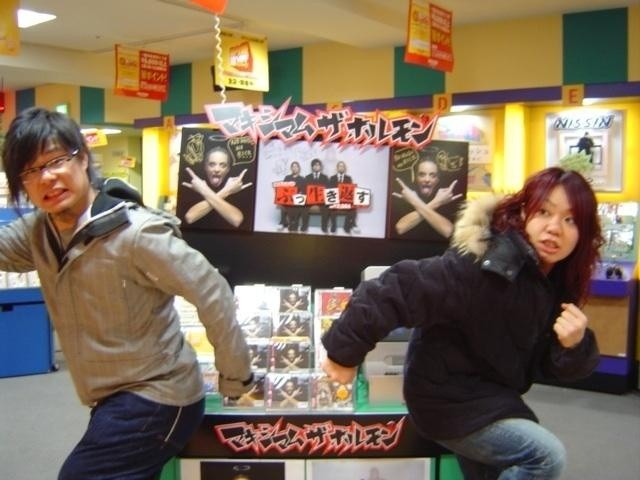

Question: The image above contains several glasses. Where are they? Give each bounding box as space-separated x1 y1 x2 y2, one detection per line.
18 148 80 183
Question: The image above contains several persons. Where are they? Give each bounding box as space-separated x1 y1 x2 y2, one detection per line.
0 107 255 479
277 159 360 235
391 157 463 238
225 290 307 409
322 167 604 480
577 131 594 155
181 145 254 231
